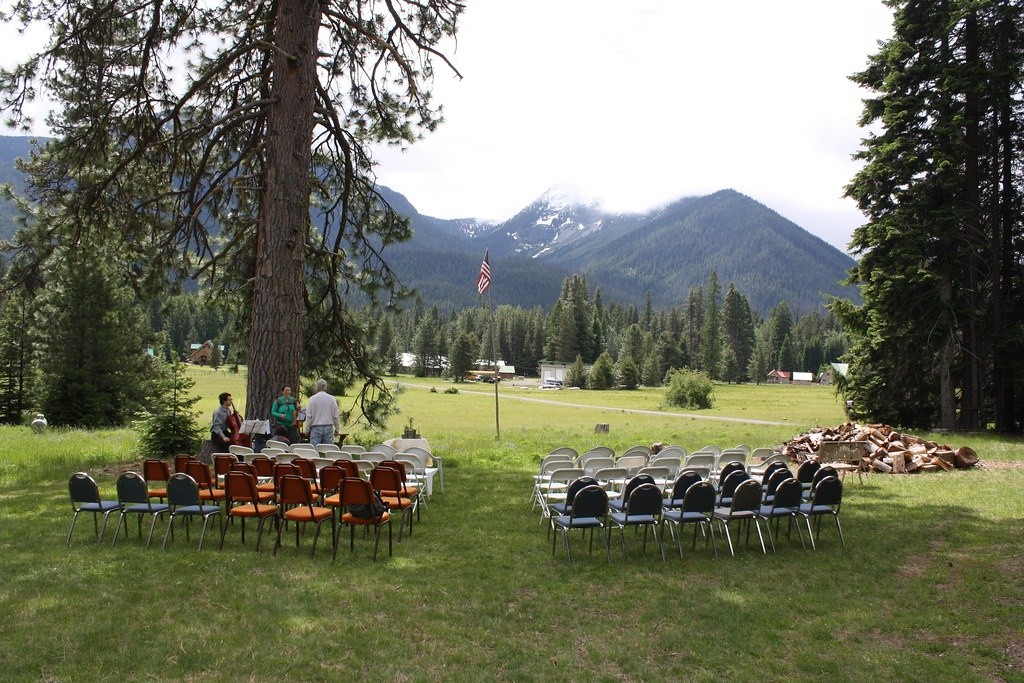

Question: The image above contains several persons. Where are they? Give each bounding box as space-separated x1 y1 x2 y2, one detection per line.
210 393 244 461
304 378 339 447
270 386 301 443
270 426 291 446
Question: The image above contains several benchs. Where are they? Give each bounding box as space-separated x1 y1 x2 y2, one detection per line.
806 440 866 485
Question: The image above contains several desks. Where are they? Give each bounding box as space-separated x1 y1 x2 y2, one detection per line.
383 438 434 468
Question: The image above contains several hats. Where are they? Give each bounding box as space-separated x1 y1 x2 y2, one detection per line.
276 426 288 436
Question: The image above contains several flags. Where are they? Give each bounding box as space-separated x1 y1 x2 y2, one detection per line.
477 250 491 294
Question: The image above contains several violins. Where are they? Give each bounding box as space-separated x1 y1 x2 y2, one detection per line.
292 399 302 429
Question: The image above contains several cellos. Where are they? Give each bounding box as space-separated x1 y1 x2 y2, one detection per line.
227 401 251 449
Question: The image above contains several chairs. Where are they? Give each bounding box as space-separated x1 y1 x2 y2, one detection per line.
68 440 444 566
529 445 847 567
